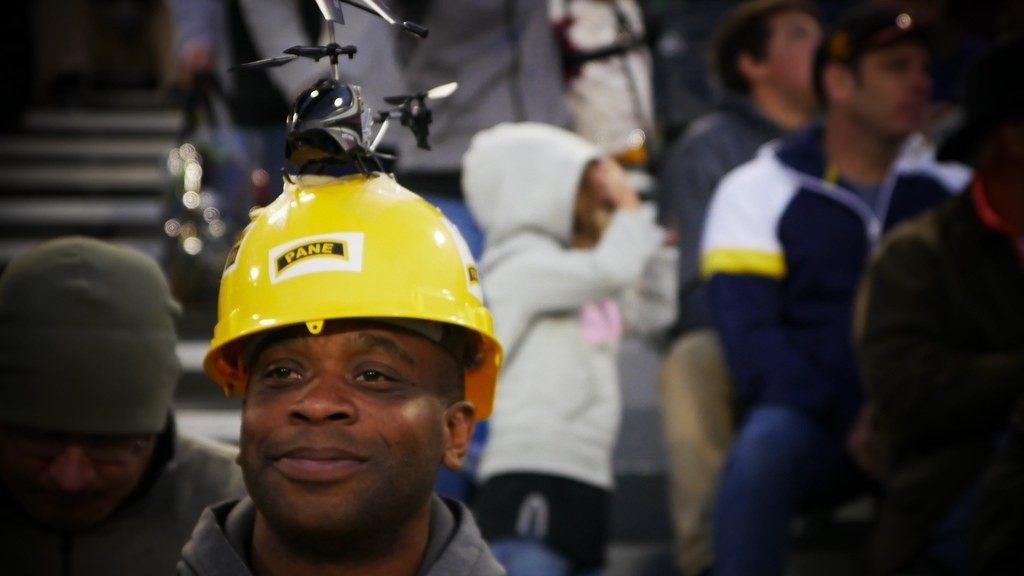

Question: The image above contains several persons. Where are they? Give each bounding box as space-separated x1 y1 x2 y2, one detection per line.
0 235 252 575
461 121 680 574
158 2 225 146
655 2 1024 576
547 1 667 163
177 173 504 574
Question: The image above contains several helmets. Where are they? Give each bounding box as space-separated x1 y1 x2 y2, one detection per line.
203 170 503 421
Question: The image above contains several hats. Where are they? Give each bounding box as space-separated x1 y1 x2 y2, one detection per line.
0 235 183 434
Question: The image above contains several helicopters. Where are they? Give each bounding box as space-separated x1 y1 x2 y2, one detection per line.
229 0 457 173
